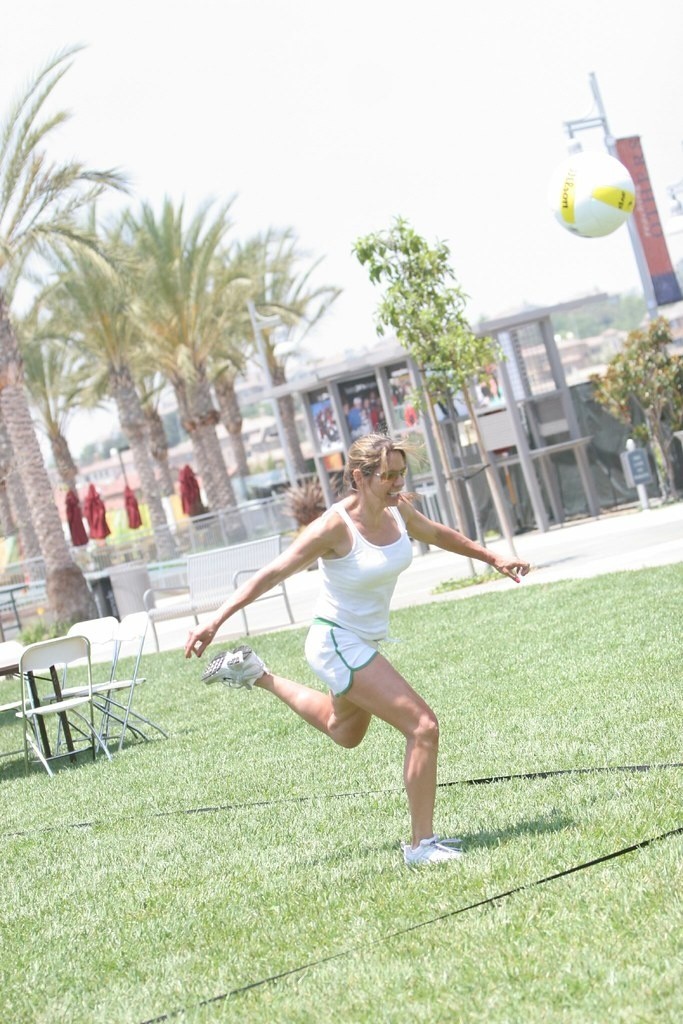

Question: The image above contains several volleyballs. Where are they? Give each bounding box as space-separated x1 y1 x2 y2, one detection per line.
550 149 638 238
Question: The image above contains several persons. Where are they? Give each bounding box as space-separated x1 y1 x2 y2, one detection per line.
347 391 388 434
317 407 338 440
184 433 530 863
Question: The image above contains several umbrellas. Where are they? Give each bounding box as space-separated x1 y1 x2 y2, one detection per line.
125 486 142 529
65 491 88 547
84 484 111 539
179 465 201 514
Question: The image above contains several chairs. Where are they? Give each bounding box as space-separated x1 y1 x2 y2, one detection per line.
0 611 168 778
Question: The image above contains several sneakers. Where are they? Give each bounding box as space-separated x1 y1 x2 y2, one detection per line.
201 646 267 690
399 836 466 866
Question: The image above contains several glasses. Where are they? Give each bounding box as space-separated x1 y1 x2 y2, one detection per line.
362 468 406 482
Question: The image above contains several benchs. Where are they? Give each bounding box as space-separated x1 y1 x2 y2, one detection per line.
144 534 293 651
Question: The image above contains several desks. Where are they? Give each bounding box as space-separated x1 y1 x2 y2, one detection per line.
0 663 77 763
